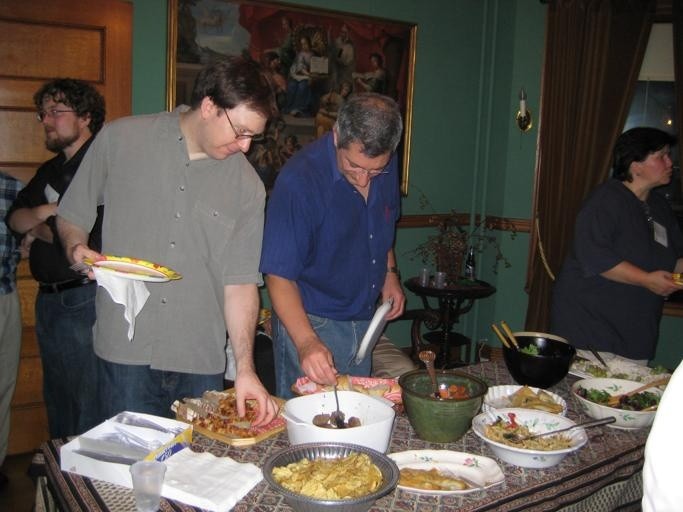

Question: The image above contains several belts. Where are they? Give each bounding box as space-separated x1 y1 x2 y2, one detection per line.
39 276 97 294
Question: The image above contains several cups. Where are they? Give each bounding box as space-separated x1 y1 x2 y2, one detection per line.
419 267 431 287
130 460 166 512
434 271 446 289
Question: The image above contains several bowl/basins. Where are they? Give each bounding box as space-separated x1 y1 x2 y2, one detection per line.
501 338 576 386
397 369 484 443
467 407 589 467
262 443 399 496
570 379 663 432
482 385 572 417
281 390 396 456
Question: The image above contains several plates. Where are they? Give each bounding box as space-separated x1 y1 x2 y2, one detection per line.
387 448 508 494
296 374 402 414
181 386 289 448
85 255 184 284
567 350 655 382
354 299 393 365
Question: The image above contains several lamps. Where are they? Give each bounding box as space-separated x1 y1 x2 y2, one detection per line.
516 88 531 130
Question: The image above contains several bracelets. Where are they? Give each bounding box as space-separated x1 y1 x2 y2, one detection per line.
387 267 401 279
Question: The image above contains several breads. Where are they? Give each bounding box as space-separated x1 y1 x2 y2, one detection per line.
398 468 468 490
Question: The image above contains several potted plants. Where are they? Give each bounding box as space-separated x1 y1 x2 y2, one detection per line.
405 184 516 280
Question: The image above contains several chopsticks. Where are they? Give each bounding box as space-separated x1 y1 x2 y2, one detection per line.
490 320 520 351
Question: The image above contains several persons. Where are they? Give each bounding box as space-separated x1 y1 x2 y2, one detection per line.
641 360 683 512
252 17 391 180
549 127 683 368
4 78 105 438
259 92 408 402
55 58 280 432
0 170 28 489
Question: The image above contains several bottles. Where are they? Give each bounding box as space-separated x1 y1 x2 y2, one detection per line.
464 245 476 281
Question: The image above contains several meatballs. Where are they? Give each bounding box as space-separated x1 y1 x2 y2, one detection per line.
312 411 361 429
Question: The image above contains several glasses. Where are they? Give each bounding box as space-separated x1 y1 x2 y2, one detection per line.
223 108 265 141
340 148 389 176
36 108 77 123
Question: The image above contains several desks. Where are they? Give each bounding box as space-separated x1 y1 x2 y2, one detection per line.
404 277 497 371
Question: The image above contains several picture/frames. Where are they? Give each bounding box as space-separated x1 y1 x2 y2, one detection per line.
167 1 418 196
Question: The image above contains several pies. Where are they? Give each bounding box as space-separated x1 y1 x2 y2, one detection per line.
505 384 564 414
